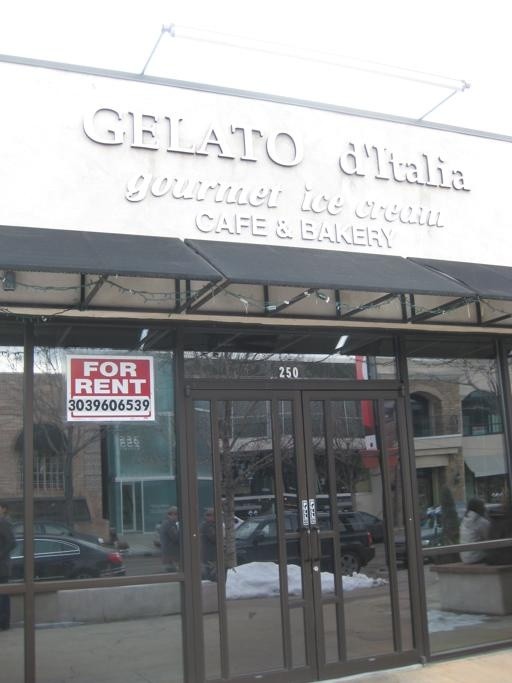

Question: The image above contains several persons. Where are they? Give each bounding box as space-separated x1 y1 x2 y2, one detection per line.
199 506 217 580
158 505 181 571
0 500 17 630
457 497 501 564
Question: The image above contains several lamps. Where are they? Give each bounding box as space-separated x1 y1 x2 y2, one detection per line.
316 288 330 303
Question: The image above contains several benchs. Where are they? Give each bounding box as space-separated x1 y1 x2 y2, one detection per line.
429 561 512 616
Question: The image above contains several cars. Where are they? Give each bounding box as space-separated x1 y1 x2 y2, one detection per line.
7 534 127 581
11 521 131 555
222 509 377 574
394 501 506 564
356 511 386 542
